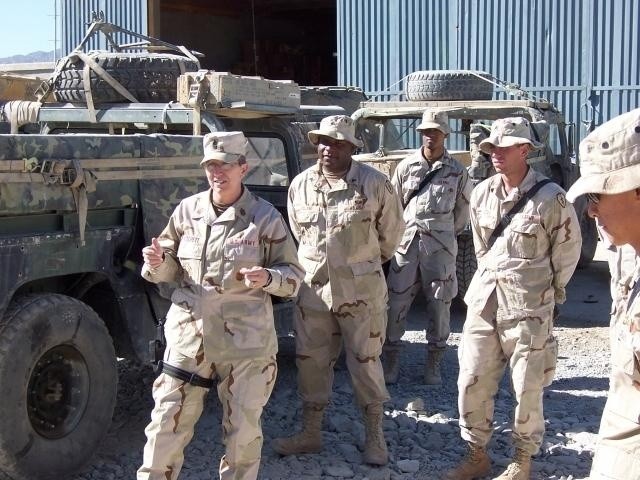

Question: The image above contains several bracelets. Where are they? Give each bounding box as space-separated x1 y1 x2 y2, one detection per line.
262 269 272 288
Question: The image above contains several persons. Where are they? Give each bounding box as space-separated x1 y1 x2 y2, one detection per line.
379 108 473 386
136 131 306 480
269 115 407 467
565 107 640 479
445 117 583 480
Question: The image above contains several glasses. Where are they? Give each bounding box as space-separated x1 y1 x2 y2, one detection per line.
584 193 600 204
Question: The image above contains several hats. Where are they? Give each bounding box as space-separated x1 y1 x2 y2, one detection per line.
415 110 450 135
564 107 640 204
307 115 365 149
479 117 544 155
199 130 248 166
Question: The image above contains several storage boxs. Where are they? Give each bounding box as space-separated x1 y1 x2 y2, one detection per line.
177 70 303 109
0 70 54 102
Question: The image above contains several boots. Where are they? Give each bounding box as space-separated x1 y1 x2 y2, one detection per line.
362 414 389 466
382 351 400 384
446 442 489 480
269 410 324 456
423 351 443 385
491 447 531 480
